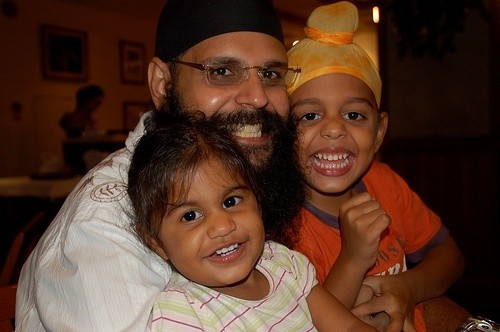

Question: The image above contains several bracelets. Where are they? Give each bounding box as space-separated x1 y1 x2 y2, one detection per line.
454 314 500 332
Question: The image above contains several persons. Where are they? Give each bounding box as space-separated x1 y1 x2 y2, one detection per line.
14 0 500 332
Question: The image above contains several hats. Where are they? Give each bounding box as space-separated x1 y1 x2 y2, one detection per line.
286 2 384 110
153 0 286 64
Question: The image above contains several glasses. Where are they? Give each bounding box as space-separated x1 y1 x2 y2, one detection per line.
173 58 302 89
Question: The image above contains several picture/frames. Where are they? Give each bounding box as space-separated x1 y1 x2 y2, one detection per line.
122 100 153 133
119 39 147 83
40 23 89 81
